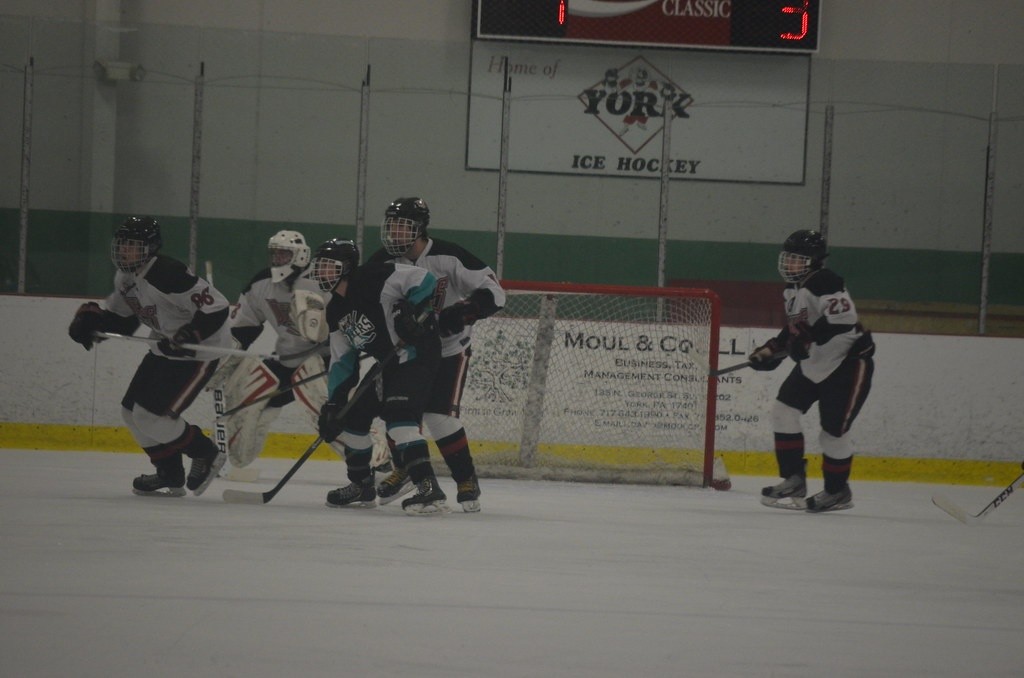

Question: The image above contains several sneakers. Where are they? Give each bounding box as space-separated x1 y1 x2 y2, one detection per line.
402 475 449 516
132 451 186 497
450 457 481 512
325 467 378 509
376 458 417 505
762 459 809 510
803 481 855 514
185 437 227 497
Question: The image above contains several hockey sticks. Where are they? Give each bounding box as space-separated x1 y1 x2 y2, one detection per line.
192 259 230 497
94 329 327 361
686 346 789 377
221 306 433 505
224 353 371 418
931 472 1024 527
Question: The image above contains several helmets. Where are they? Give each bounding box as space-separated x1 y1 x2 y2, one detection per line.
784 231 825 271
309 239 360 294
110 216 163 273
267 230 310 283
380 197 430 257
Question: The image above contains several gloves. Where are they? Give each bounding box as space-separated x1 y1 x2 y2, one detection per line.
748 336 789 371
786 322 815 365
438 295 488 338
392 299 425 342
157 324 202 357
69 302 105 349
317 399 356 444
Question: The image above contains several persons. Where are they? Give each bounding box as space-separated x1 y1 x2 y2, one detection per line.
312 240 453 516
69 216 230 497
360 197 507 513
750 228 876 513
229 230 347 481
